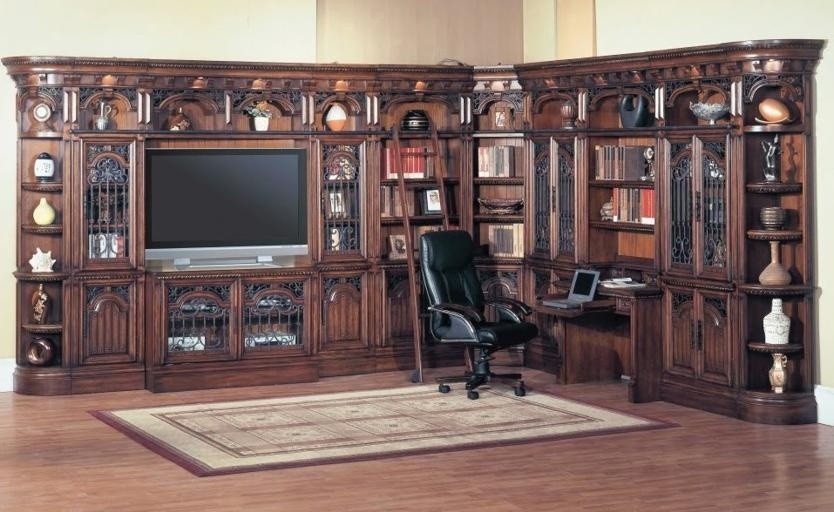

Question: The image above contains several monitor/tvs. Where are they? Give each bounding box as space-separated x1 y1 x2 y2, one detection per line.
144 147 309 270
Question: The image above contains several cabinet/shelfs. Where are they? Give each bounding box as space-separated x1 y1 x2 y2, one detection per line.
465 62 529 370
2 55 466 398
514 38 826 424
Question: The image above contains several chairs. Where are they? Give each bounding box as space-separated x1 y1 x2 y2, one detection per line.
417 230 540 400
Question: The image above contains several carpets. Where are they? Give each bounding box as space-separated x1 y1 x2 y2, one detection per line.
84 379 678 479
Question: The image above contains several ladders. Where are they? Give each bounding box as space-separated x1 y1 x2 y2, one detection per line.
391 124 474 383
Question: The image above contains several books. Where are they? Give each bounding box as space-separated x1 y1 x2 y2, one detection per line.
487 222 525 260
592 143 655 226
476 145 523 179
380 145 459 262
596 277 647 289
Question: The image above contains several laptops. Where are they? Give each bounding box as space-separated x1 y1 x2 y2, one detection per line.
542 268 601 309
